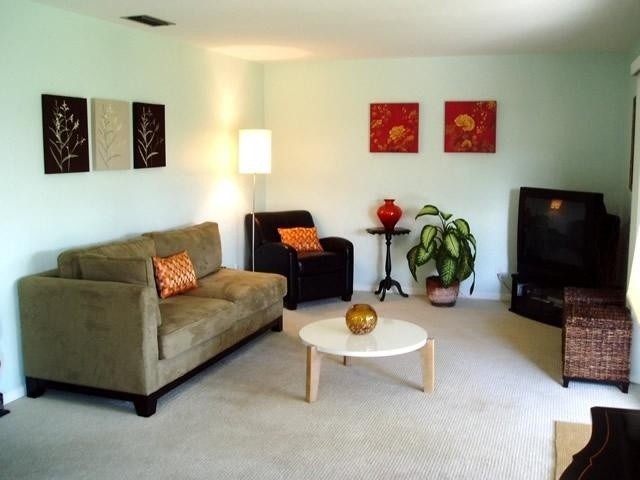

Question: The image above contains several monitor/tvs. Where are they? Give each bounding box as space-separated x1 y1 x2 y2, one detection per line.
516 186 621 289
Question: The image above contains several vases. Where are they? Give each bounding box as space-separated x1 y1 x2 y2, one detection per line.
378 199 402 229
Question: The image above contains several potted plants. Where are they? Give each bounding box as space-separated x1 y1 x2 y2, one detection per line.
406 205 478 307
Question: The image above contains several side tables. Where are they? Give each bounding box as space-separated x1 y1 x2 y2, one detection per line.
368 227 409 302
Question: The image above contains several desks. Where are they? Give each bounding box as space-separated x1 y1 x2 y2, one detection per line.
564 306 632 393
559 407 640 480
562 287 624 315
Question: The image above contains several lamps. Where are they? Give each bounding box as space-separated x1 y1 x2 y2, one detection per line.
239 128 272 273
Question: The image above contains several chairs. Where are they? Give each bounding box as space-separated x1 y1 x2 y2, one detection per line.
246 210 354 309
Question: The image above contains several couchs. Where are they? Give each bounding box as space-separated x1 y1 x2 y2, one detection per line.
16 222 288 417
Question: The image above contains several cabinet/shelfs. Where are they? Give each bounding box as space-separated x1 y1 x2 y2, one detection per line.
509 273 616 329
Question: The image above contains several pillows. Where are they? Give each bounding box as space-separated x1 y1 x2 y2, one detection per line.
142 222 222 285
77 254 162 328
57 237 157 280
277 228 323 256
152 251 199 298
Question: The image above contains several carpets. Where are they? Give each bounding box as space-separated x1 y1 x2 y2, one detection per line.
554 422 592 479
